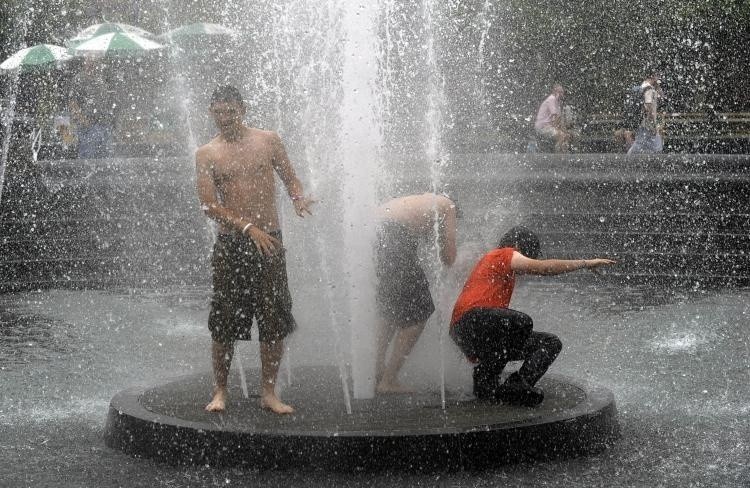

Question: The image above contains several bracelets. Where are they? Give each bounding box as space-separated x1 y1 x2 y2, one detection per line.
241 223 253 234
291 192 302 201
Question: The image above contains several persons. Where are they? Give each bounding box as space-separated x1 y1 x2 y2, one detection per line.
625 61 664 153
195 85 318 414
371 193 458 394
448 225 618 406
533 83 566 153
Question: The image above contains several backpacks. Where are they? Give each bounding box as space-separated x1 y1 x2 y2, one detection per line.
619 84 651 130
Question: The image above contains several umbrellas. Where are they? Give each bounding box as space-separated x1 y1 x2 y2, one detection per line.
0 20 236 70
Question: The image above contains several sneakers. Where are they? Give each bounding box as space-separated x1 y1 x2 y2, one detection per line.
499 371 544 406
473 364 497 398
